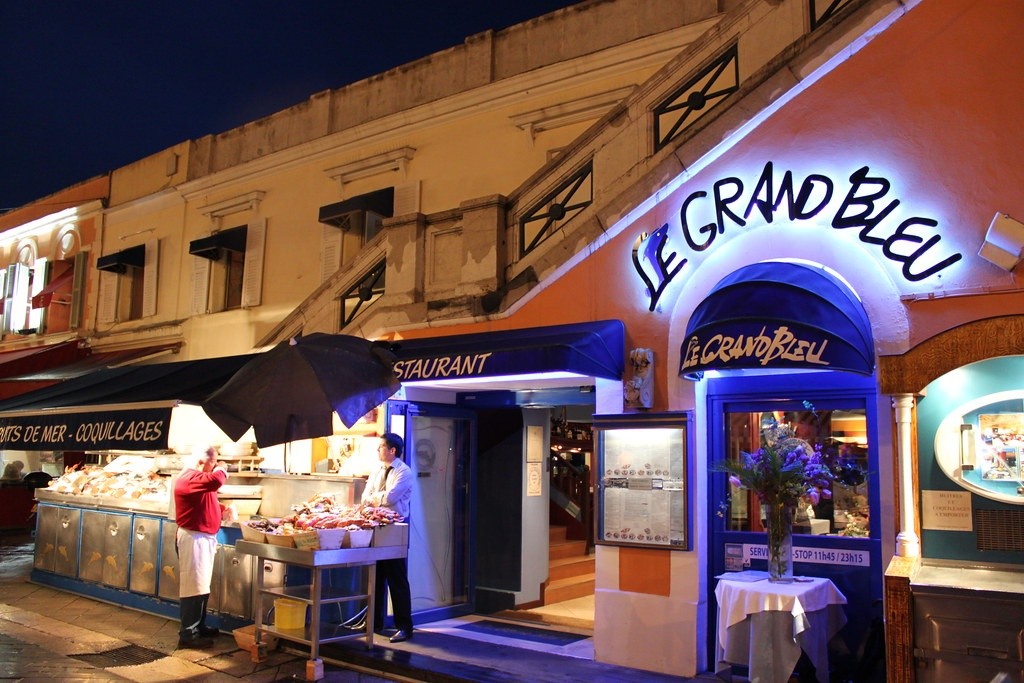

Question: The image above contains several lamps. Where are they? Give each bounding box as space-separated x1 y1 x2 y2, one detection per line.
977 212 1024 271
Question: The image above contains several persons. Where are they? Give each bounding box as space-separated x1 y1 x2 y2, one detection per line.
360 433 414 643
0 460 27 480
174 447 230 649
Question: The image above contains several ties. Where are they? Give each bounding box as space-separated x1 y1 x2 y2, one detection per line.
379 466 393 492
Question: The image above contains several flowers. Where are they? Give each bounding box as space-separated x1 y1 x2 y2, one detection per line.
711 400 847 578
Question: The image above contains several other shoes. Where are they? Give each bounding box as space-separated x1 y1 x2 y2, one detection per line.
200 627 219 637
178 638 213 649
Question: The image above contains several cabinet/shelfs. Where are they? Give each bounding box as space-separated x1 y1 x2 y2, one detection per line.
234 539 407 661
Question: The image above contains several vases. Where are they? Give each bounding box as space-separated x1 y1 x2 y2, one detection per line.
232 625 277 653
755 494 796 584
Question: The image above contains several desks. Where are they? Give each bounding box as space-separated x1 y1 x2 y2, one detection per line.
713 570 847 683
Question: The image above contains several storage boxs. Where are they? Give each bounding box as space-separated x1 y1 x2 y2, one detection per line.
370 522 408 546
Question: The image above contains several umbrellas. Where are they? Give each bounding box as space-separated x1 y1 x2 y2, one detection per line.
200 331 402 474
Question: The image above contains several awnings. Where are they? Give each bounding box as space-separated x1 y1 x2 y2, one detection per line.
0 338 264 456
677 258 876 382
391 319 625 382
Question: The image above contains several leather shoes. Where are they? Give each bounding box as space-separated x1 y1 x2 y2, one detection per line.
389 630 412 642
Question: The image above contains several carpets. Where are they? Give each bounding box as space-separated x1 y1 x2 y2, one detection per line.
454 620 591 648
